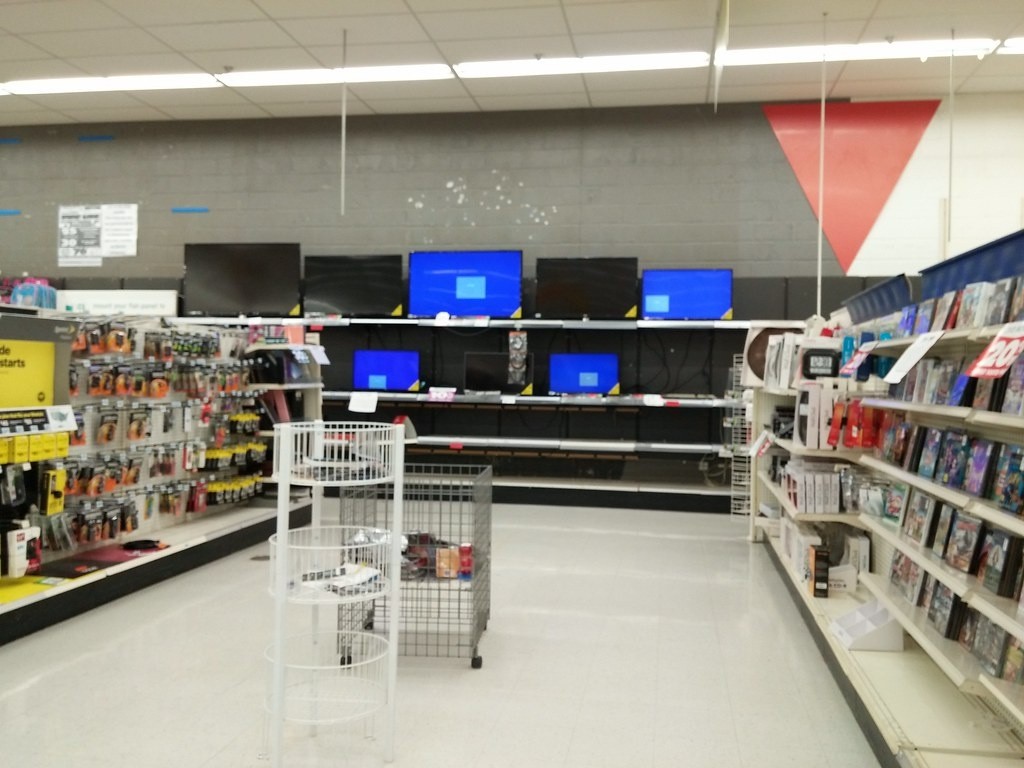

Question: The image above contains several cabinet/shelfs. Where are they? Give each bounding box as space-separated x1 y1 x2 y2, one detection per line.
745 297 1024 768
254 420 404 766
318 315 810 499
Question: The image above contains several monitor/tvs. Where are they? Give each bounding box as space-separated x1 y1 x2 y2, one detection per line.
641 269 734 320
406 250 523 319
181 241 302 317
464 352 534 395
535 258 637 319
303 254 403 317
352 349 420 391
548 353 620 395
